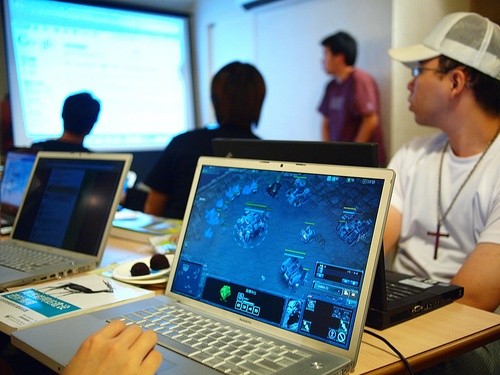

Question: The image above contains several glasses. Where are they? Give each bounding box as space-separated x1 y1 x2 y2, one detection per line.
411 63 448 78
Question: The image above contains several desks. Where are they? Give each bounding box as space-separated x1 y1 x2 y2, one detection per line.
0 209 500 375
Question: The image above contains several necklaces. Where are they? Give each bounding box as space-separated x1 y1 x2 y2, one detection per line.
427 128 500 260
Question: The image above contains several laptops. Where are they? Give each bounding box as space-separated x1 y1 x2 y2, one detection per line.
0 138 464 375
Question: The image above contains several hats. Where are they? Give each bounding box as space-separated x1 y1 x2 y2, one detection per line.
388 11 500 81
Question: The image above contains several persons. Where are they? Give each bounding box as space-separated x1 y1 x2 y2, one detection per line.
318 31 387 168
382 11 500 375
142 61 266 220
29 92 100 154
60 319 163 375
